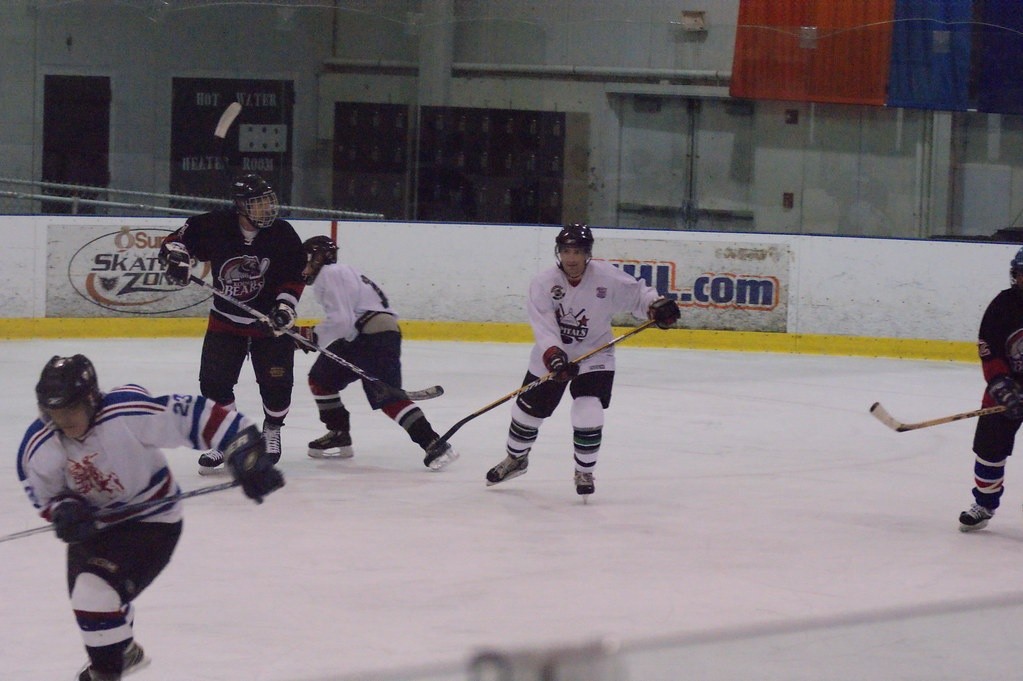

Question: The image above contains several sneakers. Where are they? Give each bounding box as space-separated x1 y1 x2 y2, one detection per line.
486 451 529 487
307 426 353 458
573 467 595 505
198 447 226 477
424 440 458 470
263 418 282 463
958 504 994 534
77 637 151 681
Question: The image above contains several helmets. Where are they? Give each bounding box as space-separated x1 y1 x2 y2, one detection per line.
231 174 279 228
555 224 595 249
296 236 339 284
36 354 101 412
1011 248 1023 272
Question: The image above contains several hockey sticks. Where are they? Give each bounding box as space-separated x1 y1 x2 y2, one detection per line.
188 274 444 400
870 401 1007 432
0 479 244 542
426 319 656 451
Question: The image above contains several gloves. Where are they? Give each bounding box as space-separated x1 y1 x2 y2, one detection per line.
164 251 190 287
268 300 297 336
542 346 580 383
55 501 94 544
226 426 285 505
988 377 1023 420
651 299 680 330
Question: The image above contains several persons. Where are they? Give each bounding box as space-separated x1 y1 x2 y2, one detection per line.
158 173 306 476
959 245 1023 532
487 223 681 503
293 235 460 472
16 354 285 681
